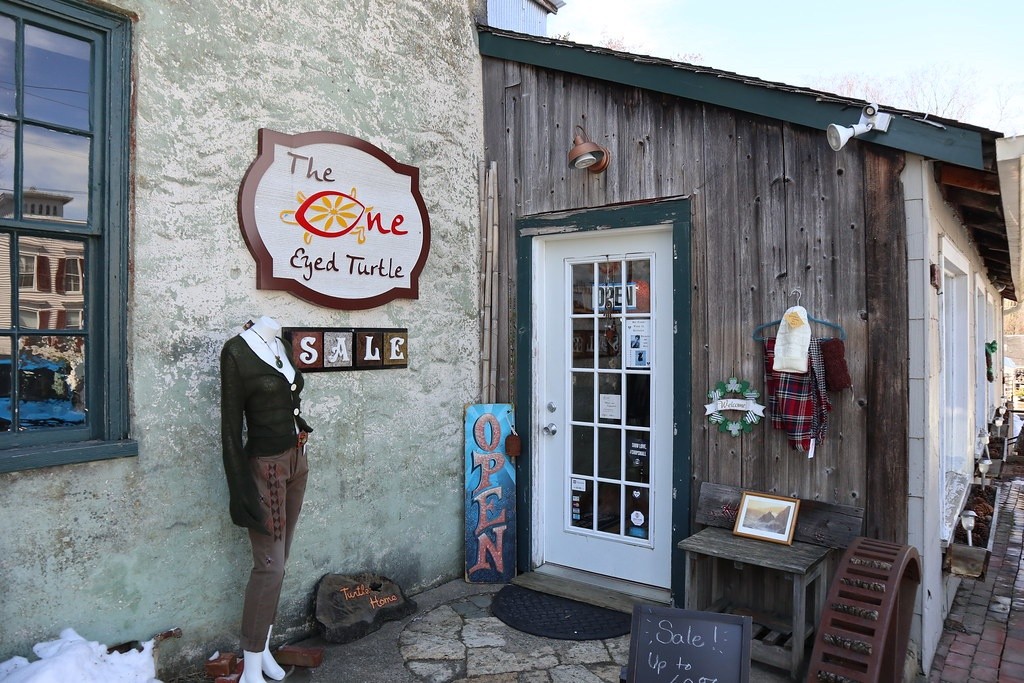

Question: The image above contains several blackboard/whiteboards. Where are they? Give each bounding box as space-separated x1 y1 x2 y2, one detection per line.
626 604 752 683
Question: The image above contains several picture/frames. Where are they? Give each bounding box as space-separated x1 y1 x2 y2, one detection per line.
732 490 802 545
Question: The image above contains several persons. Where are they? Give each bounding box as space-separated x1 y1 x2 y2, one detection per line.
631 335 640 348
220 312 313 683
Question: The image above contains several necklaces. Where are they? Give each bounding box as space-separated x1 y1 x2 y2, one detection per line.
251 327 284 369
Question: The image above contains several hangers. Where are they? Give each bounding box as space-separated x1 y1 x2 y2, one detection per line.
751 289 846 342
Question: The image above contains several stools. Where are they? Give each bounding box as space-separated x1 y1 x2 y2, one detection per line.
678 525 833 680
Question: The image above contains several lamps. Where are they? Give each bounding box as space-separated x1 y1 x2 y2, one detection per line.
567 123 611 174
826 103 893 152
